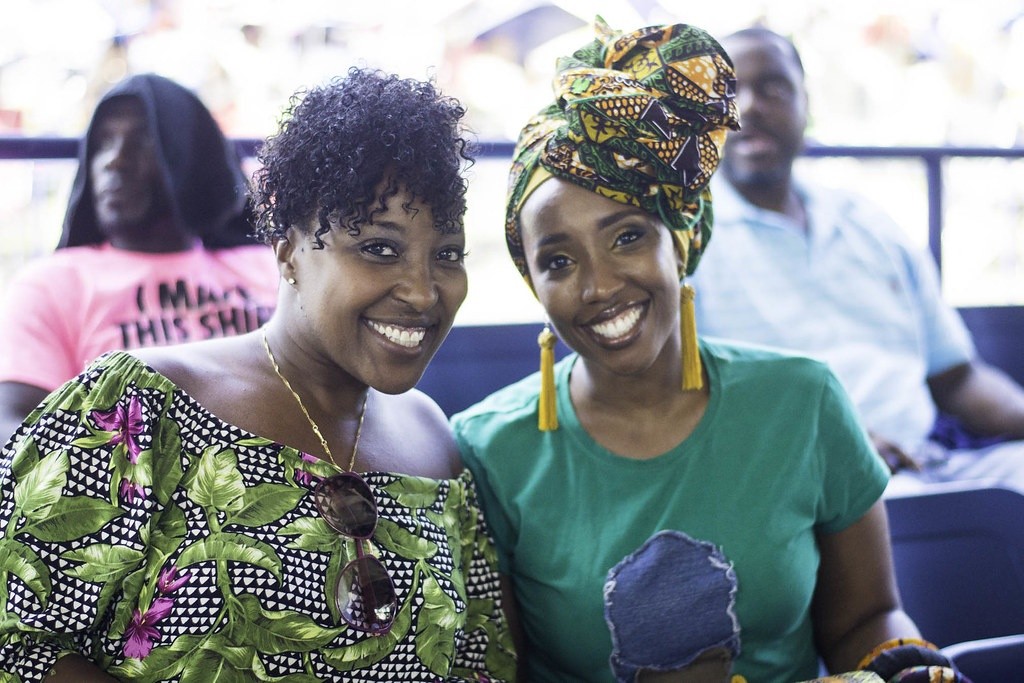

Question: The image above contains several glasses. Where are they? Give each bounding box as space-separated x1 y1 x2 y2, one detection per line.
314 471 398 635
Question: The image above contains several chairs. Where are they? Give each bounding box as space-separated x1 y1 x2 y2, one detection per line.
930 305 1022 451
417 322 572 420
819 483 1024 683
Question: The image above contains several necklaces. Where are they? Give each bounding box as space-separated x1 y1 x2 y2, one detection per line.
259 322 371 473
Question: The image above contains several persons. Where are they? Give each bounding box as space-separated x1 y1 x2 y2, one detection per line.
1 63 519 683
680 28 1024 683
0 73 282 447
450 16 967 683
